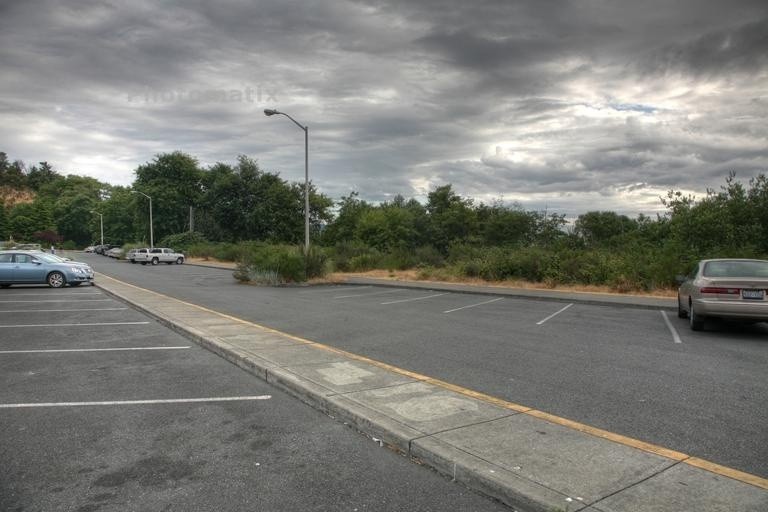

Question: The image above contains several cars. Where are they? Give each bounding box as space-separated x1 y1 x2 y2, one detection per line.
674 256 767 332
0 242 185 289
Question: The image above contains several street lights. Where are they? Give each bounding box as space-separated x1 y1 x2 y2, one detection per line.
129 189 153 249
88 209 104 245
262 107 310 252
